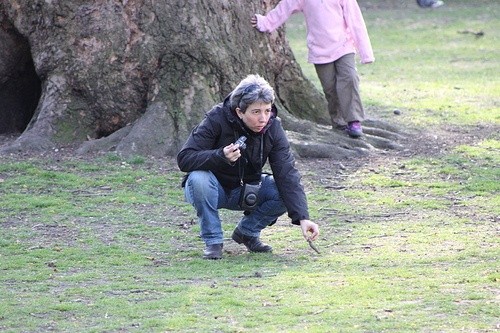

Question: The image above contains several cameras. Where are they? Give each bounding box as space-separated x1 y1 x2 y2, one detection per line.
233 136 247 149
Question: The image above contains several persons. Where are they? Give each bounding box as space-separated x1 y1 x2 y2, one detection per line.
176 74 320 260
250 0 375 138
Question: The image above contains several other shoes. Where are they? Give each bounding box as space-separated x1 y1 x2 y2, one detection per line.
431 0 443 8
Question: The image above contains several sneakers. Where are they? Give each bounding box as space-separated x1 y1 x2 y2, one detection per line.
345 121 361 136
203 243 223 260
339 125 347 130
231 225 273 253
